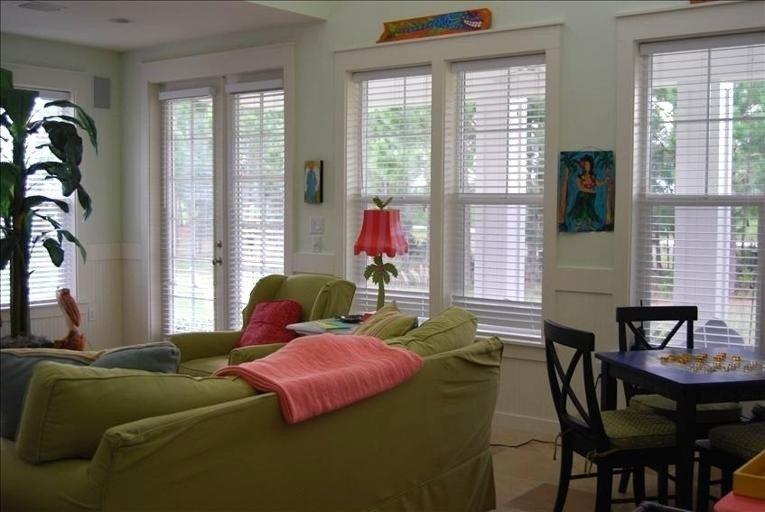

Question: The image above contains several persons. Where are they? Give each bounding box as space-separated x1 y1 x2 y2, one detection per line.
565 154 609 232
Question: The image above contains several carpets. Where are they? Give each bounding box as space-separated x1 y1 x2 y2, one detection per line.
497 477 634 511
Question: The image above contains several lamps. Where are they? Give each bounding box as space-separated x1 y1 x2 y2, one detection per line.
349 192 412 318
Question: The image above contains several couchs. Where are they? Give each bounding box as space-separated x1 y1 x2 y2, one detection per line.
0 300 505 512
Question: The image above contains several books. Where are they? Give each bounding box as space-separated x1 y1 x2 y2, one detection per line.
284 318 353 335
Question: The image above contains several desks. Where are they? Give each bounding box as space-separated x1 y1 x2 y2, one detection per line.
594 342 765 511
283 304 373 338
717 461 765 510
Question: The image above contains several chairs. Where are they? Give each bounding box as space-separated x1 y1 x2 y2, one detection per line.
162 269 357 381
539 314 698 512
614 298 741 509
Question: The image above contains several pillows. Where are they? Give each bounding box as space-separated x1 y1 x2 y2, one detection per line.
239 296 302 347
347 300 421 340
0 338 185 441
